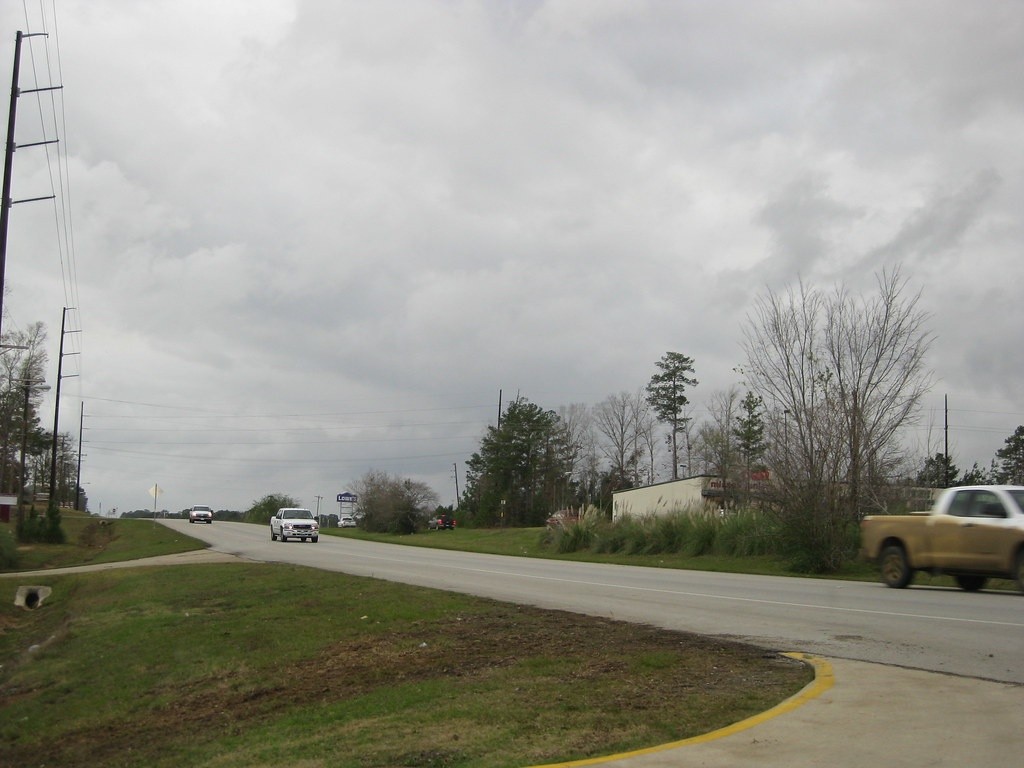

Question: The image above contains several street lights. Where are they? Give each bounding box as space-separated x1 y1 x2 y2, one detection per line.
783 408 791 475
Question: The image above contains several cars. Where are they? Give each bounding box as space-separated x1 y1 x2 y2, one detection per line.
427 514 456 531
546 510 584 530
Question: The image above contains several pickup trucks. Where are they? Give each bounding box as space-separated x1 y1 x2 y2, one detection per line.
337 517 356 528
861 484 1024 592
269 508 319 543
189 505 212 524
